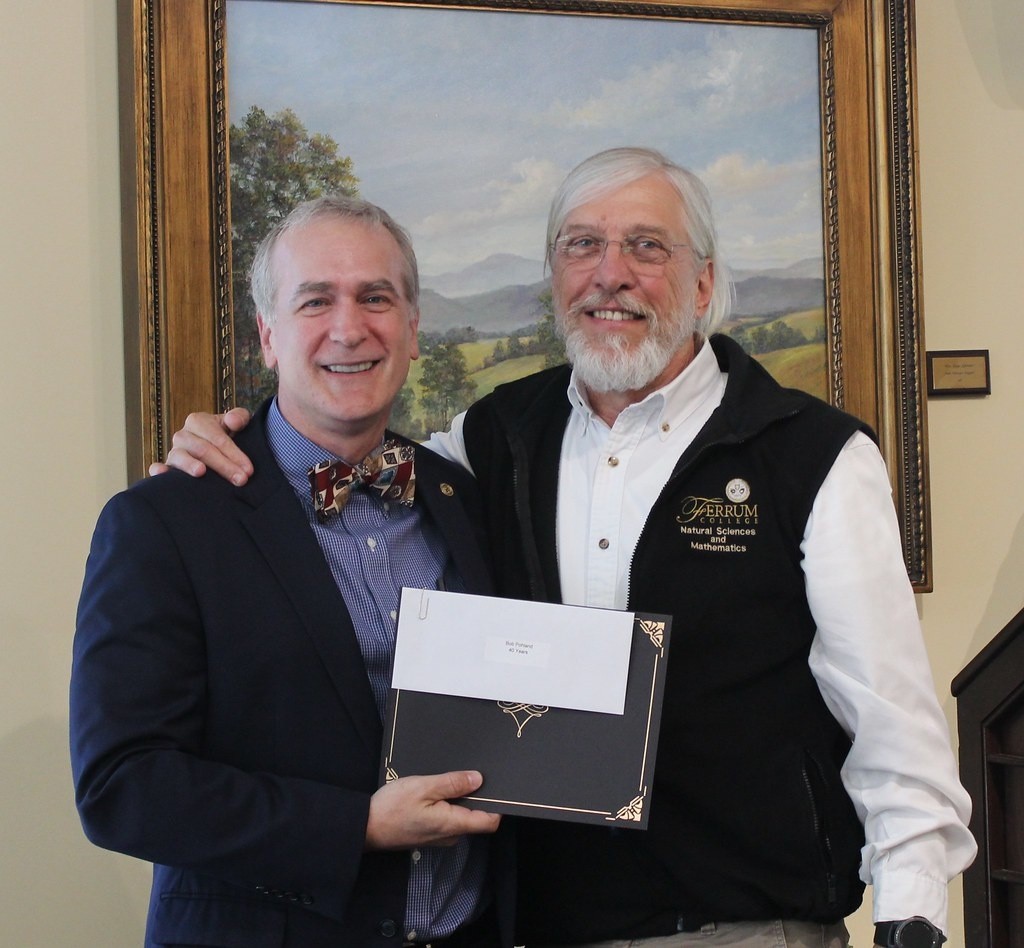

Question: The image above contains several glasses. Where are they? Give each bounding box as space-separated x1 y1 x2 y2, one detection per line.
554 234 697 266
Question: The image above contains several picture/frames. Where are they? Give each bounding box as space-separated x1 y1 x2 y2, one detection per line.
131 0 933 594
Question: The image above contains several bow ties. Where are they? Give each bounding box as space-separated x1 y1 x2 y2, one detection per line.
307 438 414 527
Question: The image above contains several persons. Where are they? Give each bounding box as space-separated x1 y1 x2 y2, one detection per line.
69 195 530 948
150 147 978 948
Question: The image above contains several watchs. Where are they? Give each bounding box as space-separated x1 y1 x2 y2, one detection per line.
871 915 948 947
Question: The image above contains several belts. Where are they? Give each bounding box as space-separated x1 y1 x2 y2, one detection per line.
614 906 735 935
